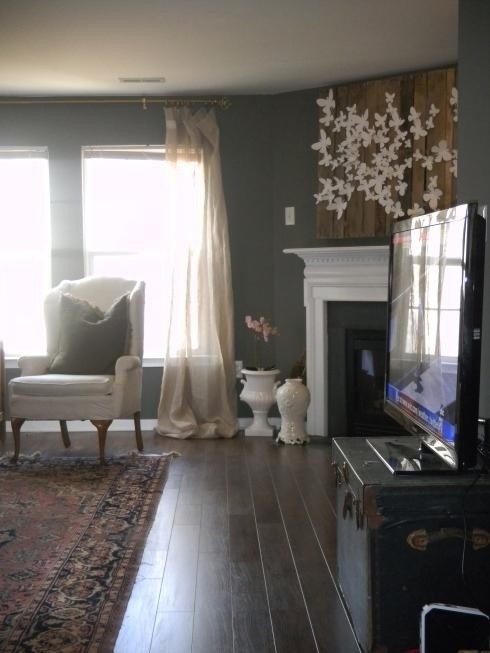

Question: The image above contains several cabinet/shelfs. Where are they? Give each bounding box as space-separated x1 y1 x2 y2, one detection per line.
329 435 490 653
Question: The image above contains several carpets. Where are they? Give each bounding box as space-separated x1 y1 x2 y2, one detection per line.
0 451 183 653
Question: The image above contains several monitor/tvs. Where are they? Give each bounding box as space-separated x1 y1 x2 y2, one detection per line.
365 201 487 475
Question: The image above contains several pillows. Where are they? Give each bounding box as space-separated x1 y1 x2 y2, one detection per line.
46 292 133 375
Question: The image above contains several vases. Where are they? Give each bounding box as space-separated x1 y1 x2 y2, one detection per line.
239 370 280 437
275 378 312 446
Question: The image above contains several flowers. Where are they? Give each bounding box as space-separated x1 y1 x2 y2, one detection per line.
245 315 280 372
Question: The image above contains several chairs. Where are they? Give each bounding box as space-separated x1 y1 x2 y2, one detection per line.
7 275 145 467
